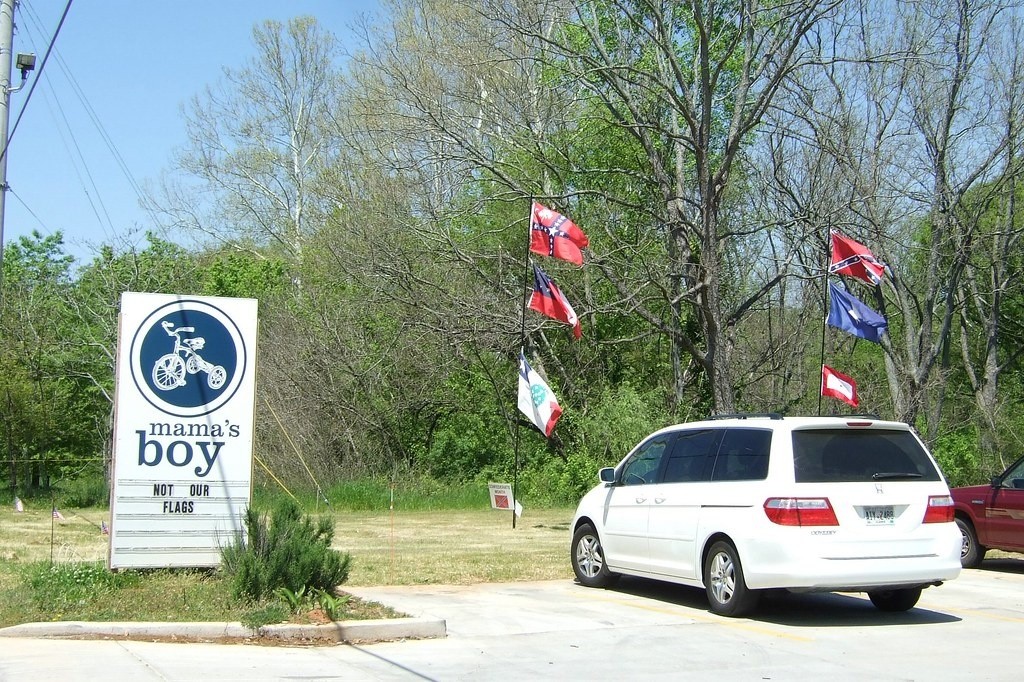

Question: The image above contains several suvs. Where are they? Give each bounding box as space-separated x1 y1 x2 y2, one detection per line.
570 413 962 618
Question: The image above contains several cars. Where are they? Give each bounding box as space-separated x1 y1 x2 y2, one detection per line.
949 454 1024 568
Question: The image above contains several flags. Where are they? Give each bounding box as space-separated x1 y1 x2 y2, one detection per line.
825 279 888 346
827 228 885 291
525 264 582 341
821 364 859 408
528 198 588 268
517 345 562 439
